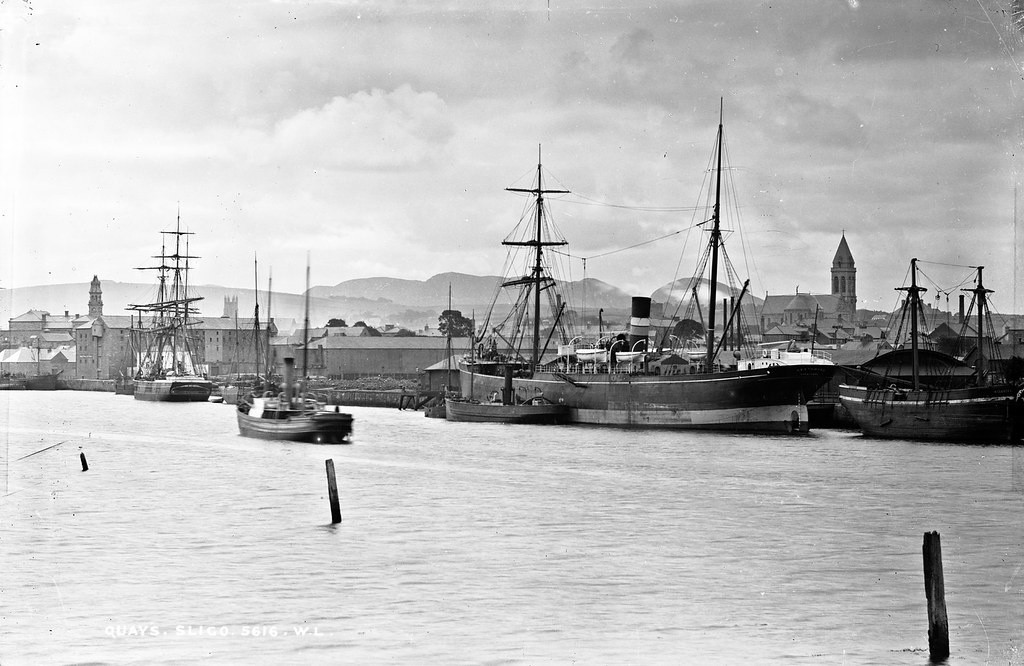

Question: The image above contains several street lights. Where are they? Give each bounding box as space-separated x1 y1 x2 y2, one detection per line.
566 335 583 375
630 338 646 374
609 338 626 374
669 334 701 352
593 335 610 374
598 307 604 348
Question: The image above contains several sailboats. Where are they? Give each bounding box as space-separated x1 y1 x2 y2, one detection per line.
114 204 221 403
222 254 293 406
838 257 1024 442
456 100 836 438
234 262 356 444
24 336 58 390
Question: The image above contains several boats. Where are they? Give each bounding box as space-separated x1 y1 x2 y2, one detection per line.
442 364 573 425
